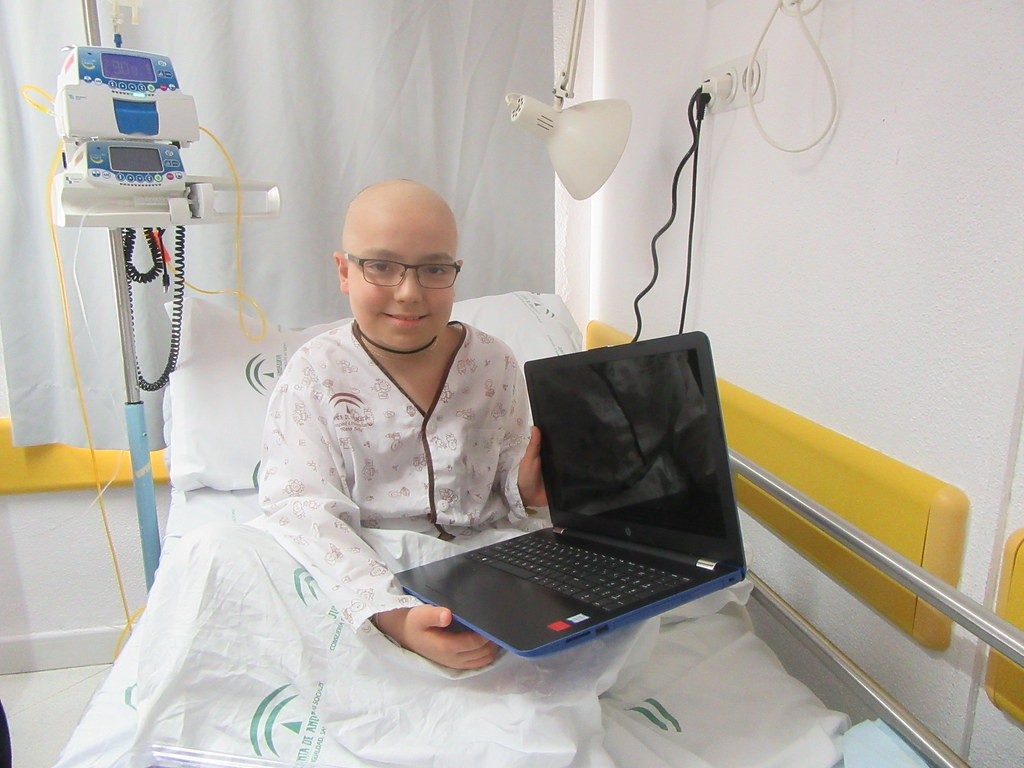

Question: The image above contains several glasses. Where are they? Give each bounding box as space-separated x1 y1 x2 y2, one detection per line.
344 253 461 290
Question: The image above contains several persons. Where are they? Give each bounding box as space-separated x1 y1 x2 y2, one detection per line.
258 179 548 670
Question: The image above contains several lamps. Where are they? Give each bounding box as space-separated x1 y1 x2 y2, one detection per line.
502 0 635 200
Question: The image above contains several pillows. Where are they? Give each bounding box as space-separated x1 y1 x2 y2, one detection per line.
167 283 583 492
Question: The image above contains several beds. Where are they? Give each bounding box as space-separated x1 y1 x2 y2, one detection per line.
52 484 1024 768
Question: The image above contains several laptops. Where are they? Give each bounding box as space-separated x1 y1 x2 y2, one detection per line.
397 331 746 657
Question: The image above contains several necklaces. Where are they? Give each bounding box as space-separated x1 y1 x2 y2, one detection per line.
358 328 438 354
